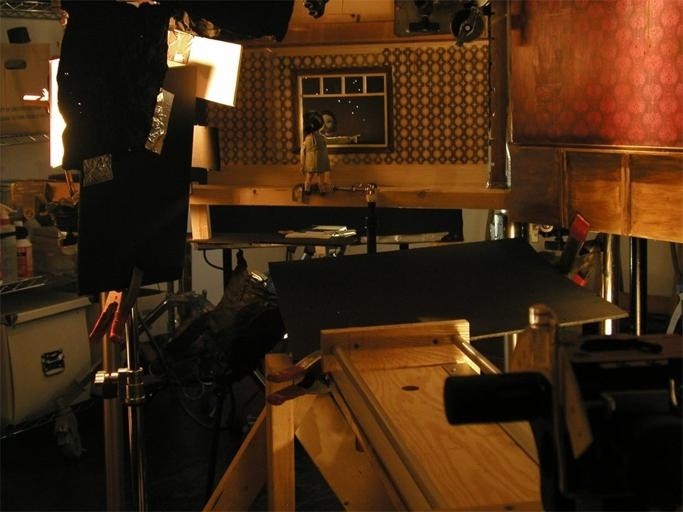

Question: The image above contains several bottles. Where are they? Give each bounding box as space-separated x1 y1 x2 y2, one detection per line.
14 226 32 280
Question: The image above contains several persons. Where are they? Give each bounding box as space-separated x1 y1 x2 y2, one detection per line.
318 112 341 135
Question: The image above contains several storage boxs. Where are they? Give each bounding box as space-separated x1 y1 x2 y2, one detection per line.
88 288 168 398
1 42 50 134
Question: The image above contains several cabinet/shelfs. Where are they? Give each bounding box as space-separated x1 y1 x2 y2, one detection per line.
1 1 61 147
186 165 510 309
